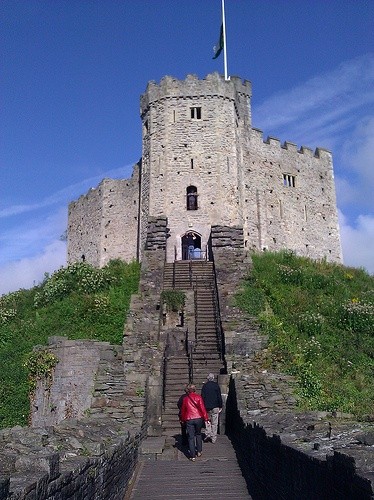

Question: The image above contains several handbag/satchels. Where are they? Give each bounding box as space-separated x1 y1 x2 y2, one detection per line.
201 422 206 428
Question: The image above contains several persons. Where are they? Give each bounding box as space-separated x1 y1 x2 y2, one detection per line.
201 372 223 444
177 389 189 452
188 189 196 209
181 383 209 462
186 231 197 260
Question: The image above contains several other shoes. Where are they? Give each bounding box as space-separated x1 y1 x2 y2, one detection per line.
204 435 211 441
190 457 195 462
198 452 202 456
212 441 215 444
